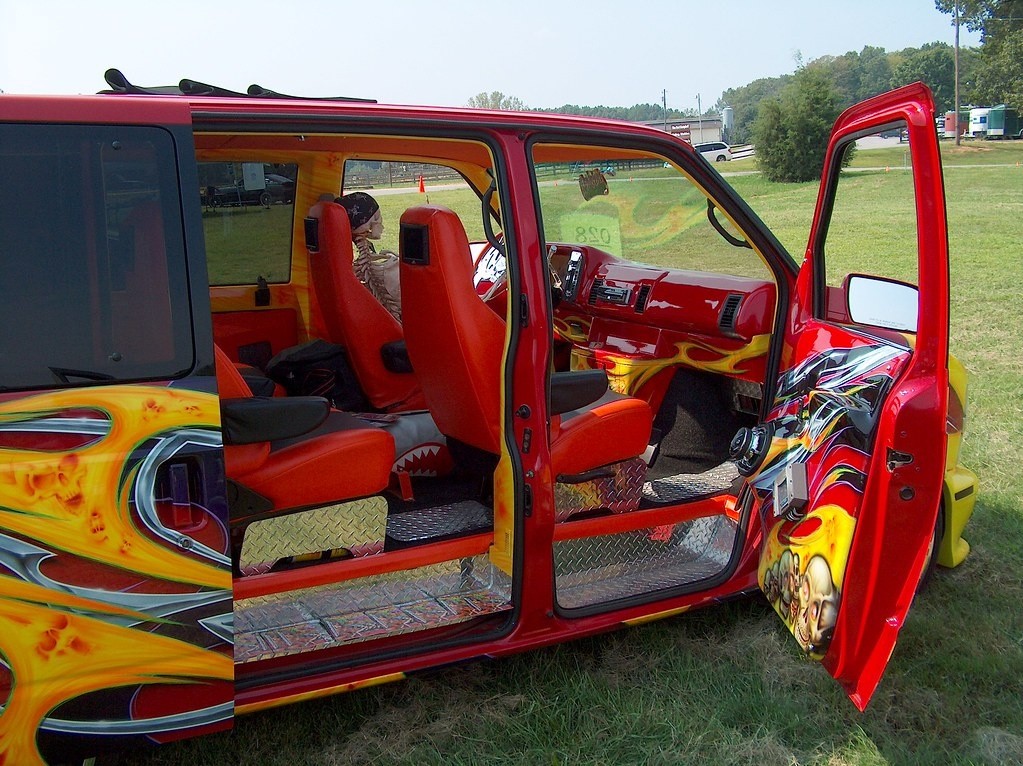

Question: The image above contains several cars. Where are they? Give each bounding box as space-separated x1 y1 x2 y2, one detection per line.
881 130 901 139
1 66 977 766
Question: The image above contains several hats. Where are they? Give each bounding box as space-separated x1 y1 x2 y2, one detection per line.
334 192 379 231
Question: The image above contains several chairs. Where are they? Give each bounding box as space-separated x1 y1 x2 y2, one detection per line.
305 200 459 503
397 207 652 517
126 217 394 520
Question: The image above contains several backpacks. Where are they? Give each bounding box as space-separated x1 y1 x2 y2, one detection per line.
266 339 372 412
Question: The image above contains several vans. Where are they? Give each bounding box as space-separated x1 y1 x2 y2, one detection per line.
663 139 732 170
